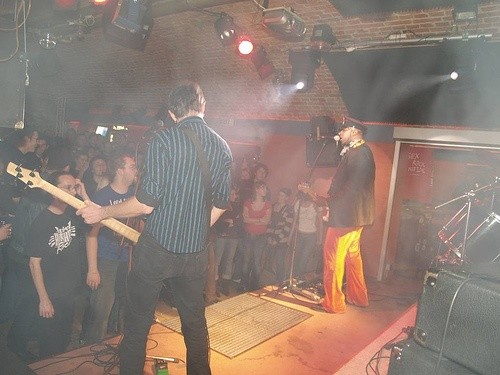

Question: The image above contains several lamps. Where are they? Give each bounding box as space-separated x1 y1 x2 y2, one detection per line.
214 15 239 47
236 37 253 57
449 67 474 82
285 47 323 93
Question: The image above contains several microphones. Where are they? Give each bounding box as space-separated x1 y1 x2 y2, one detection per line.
319 135 340 142
141 119 164 140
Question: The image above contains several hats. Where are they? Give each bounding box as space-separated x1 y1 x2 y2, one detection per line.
336 116 368 131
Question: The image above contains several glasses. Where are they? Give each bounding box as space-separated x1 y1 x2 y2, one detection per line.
59 186 79 191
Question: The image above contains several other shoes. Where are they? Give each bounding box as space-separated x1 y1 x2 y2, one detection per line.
217 279 249 297
312 304 324 311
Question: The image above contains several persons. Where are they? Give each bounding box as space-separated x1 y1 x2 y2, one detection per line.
211 156 321 300
1 123 144 364
312 117 377 313
74 81 234 375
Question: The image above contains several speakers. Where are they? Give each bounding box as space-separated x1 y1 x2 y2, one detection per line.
306 116 338 166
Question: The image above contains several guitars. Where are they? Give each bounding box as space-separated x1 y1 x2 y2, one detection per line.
5 161 140 244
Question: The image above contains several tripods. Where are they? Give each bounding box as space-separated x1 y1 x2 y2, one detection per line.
88 140 178 375
263 141 317 301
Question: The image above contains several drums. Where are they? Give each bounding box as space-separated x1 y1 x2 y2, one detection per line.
438 198 500 269
